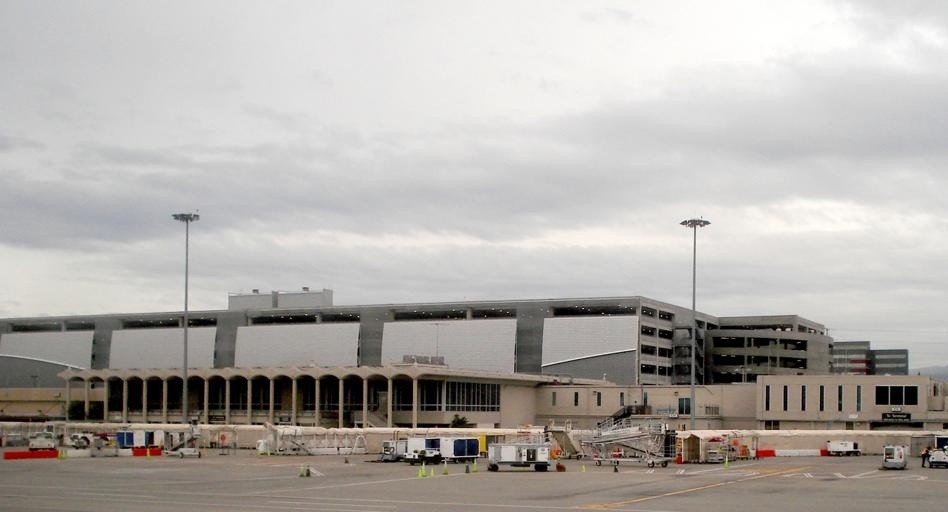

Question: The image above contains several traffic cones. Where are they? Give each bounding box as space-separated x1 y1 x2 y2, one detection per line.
420 462 427 477
472 459 478 472
724 456 730 468
442 461 448 474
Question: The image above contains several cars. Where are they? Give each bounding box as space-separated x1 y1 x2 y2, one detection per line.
29 432 60 449
927 448 948 468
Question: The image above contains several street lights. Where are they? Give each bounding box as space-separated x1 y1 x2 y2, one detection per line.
680 220 710 428
173 214 197 422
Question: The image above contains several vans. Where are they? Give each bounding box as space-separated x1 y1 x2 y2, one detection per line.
882 446 906 469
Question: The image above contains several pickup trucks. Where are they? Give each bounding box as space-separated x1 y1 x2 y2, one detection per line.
405 450 441 466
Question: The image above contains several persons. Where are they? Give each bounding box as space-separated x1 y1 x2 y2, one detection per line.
920 445 931 468
